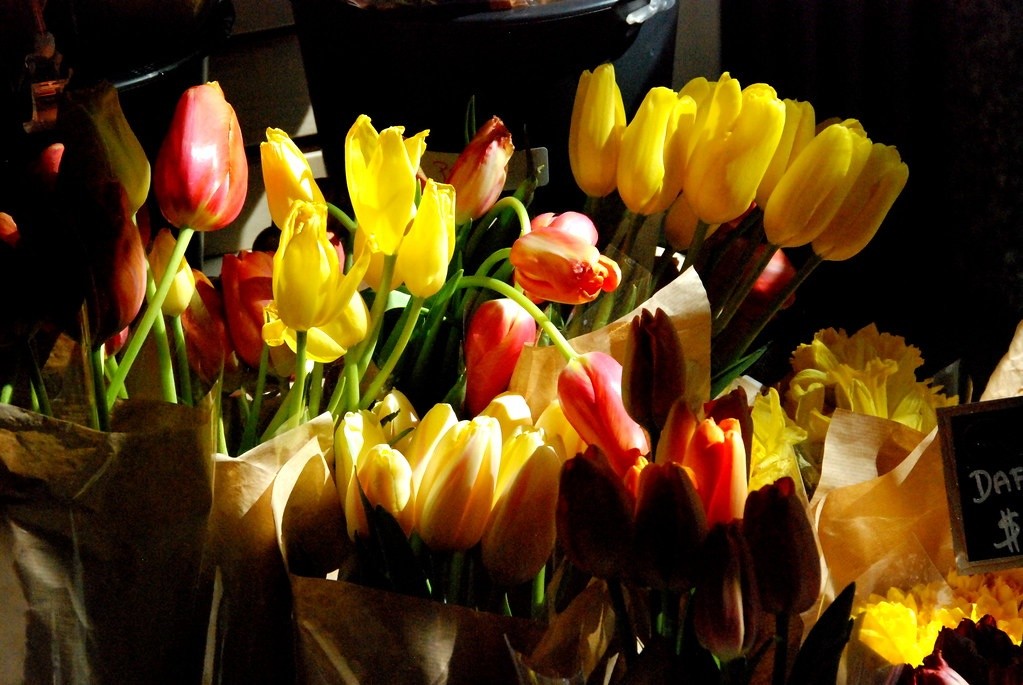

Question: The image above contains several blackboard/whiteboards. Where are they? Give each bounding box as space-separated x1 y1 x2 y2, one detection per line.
935 397 1023 569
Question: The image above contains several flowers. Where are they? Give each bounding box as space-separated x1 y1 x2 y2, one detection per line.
0 63 1023 685
